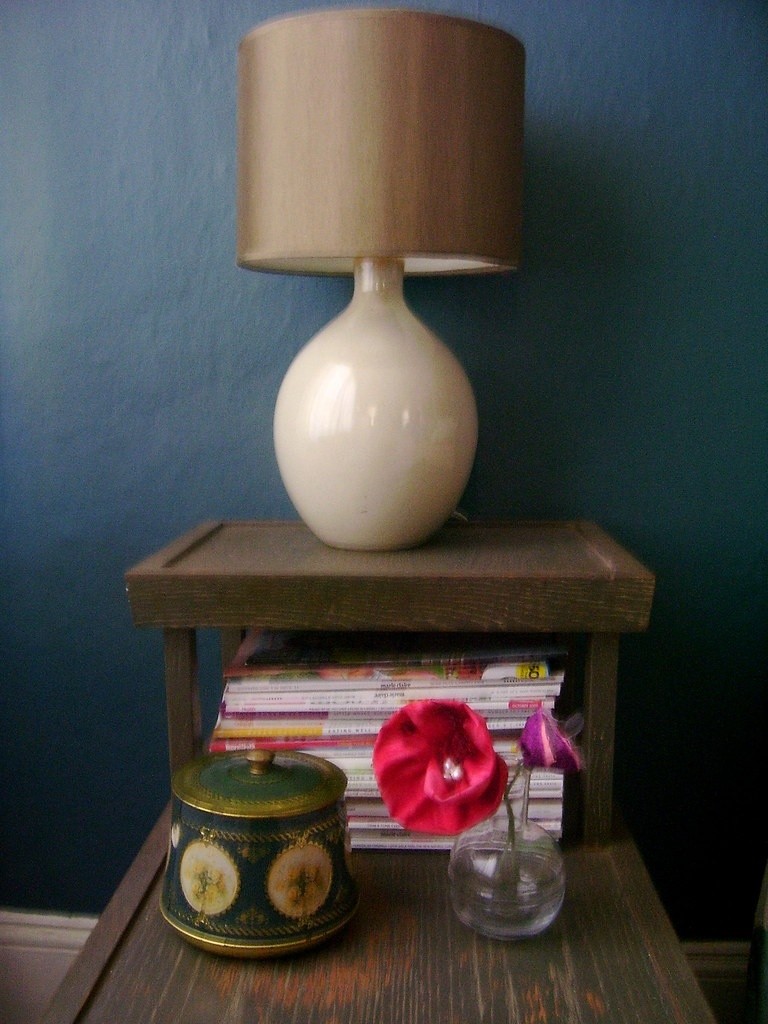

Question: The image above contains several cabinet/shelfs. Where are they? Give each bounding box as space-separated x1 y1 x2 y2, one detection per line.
42 522 718 1024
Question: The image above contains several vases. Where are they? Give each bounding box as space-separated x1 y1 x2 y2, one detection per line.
447 814 567 940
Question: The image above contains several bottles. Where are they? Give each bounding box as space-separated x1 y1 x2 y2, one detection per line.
159 749 358 958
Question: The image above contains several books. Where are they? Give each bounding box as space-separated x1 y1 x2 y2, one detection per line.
211 628 565 849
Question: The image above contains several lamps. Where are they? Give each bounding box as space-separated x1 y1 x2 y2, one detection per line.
239 6 526 553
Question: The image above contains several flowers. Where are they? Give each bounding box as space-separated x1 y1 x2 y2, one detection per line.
371 699 613 869
